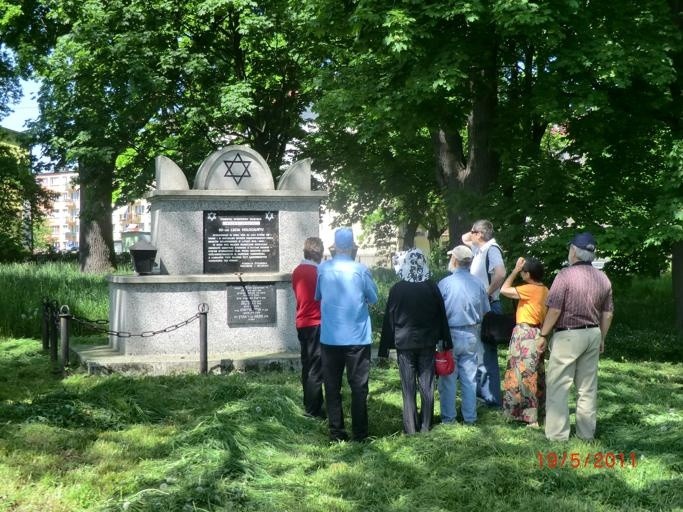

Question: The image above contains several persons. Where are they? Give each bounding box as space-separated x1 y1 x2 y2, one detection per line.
291 237 328 421
436 245 490 425
315 228 380 448
378 247 454 435
535 233 612 442
460 218 506 407
500 254 548 430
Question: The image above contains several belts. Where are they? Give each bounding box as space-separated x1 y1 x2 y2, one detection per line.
557 324 598 331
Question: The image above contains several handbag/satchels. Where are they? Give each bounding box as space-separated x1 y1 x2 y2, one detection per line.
434 351 454 375
481 311 514 344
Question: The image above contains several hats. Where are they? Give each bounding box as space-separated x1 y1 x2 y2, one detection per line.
392 248 433 283
567 232 595 251
447 245 474 263
335 228 352 245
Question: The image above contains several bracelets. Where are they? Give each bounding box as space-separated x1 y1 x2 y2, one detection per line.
538 333 550 338
